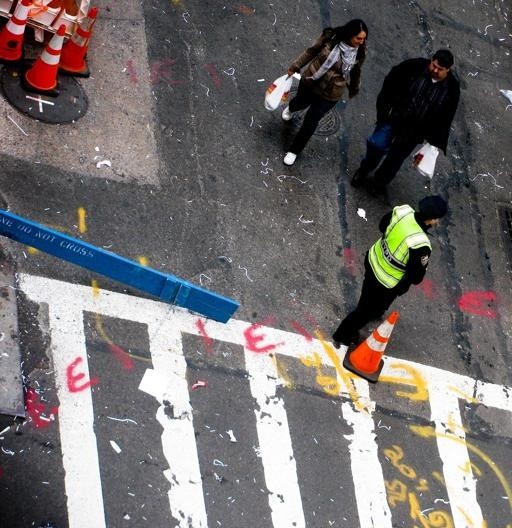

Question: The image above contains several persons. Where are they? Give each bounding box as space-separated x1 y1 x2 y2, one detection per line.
351 49 461 193
281 18 368 166
332 197 450 349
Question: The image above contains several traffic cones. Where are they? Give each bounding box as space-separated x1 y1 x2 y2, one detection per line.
343 312 399 383
0 0 98 98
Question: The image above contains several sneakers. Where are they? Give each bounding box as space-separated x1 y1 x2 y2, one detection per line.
332 332 356 346
282 105 295 120
283 152 298 166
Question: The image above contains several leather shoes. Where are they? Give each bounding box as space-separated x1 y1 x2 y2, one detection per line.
353 168 366 187
